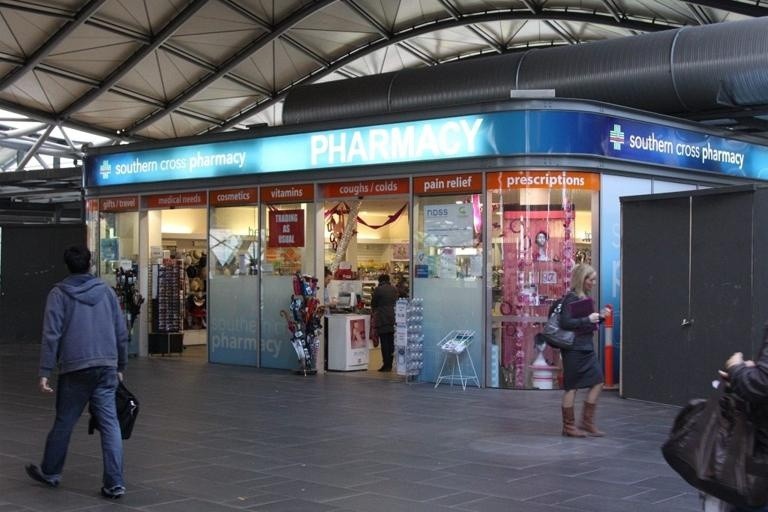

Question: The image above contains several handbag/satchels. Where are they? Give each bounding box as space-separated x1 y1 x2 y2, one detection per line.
662 399 768 512
88 381 139 440
370 311 379 348
541 308 576 351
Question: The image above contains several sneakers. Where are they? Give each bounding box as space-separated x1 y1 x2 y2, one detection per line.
25 463 58 486
378 368 389 372
102 488 124 501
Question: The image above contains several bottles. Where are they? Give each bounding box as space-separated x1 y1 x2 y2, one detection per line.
393 297 424 372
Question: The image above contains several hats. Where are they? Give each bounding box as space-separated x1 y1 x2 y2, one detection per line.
185 249 208 307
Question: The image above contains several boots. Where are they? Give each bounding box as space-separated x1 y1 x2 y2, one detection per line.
561 405 586 437
578 401 606 437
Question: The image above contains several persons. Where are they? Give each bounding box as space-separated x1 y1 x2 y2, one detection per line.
371 274 396 371
25 246 128 498
396 278 409 298
324 266 336 369
557 264 611 438
708 336 768 511
